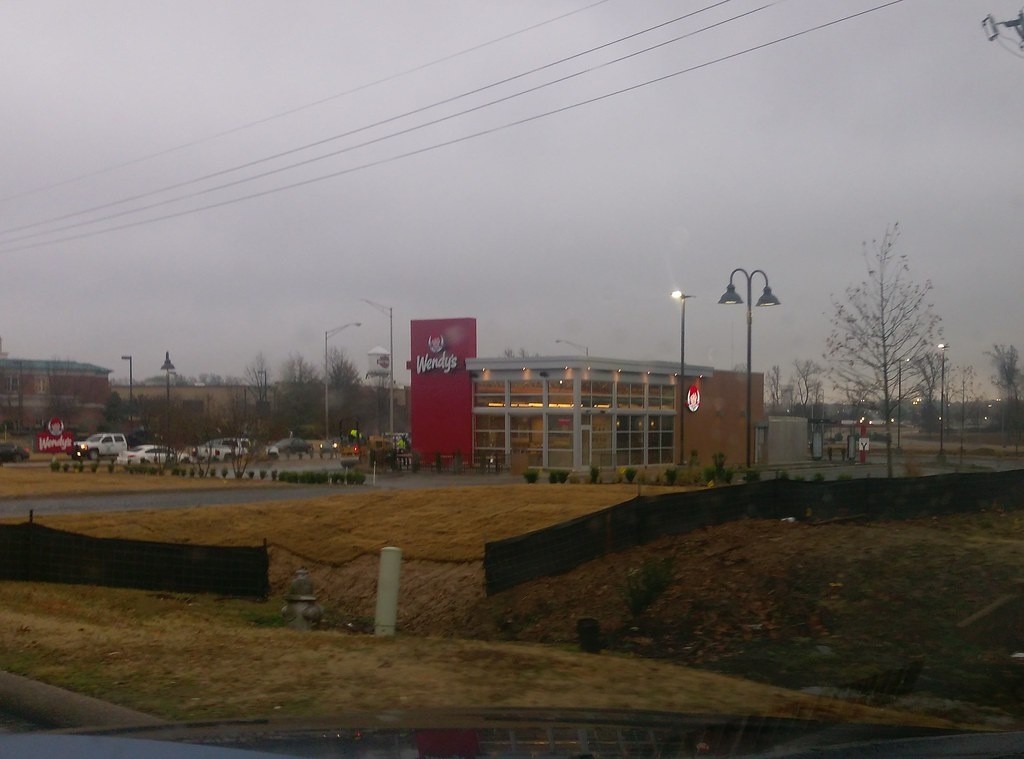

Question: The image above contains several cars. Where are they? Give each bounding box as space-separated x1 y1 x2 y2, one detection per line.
117 444 194 466
195 438 250 462
0 443 30 465
318 440 358 458
273 436 314 457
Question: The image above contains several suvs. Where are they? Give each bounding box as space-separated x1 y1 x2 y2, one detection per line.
71 434 129 461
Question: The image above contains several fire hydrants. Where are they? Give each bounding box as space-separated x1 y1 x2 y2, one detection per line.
282 567 321 631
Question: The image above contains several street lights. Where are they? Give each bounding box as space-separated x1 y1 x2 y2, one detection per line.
257 368 268 442
323 321 361 440
556 338 593 467
671 290 695 466
893 357 910 454
121 354 133 444
937 341 952 456
715 266 782 469
161 350 176 462
